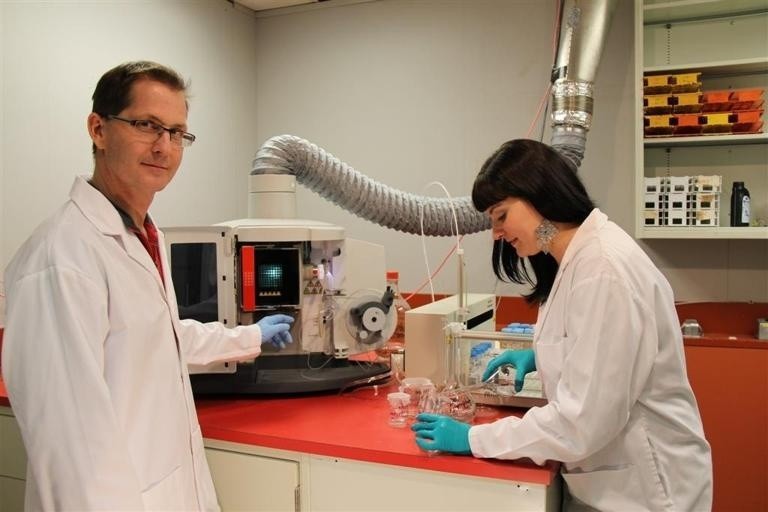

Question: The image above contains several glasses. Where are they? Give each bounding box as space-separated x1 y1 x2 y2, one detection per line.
109 116 196 150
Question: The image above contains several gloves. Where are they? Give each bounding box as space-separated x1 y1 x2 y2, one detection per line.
410 412 473 457
481 348 537 393
256 313 296 352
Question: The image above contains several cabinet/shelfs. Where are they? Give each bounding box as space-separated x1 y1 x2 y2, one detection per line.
634 0 768 238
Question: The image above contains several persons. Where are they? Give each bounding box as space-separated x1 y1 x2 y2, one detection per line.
412 139 713 512
0 62 295 512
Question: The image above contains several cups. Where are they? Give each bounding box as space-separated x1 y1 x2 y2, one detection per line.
416 393 450 454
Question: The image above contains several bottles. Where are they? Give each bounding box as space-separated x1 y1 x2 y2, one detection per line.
403 377 431 421
375 271 410 358
729 181 751 226
449 364 525 399
435 333 476 425
388 392 410 428
